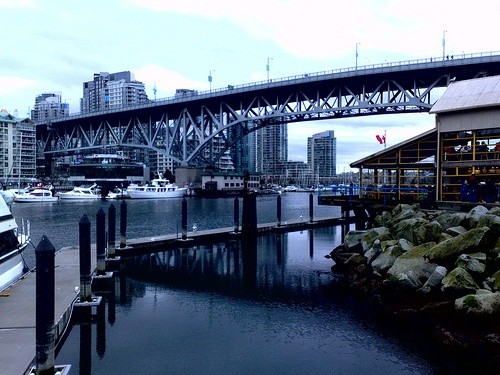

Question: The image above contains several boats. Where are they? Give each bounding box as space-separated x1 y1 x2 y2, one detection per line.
60 183 129 199
14 188 58 202
0 190 34 295
125 171 187 199
298 187 314 192
281 185 299 192
